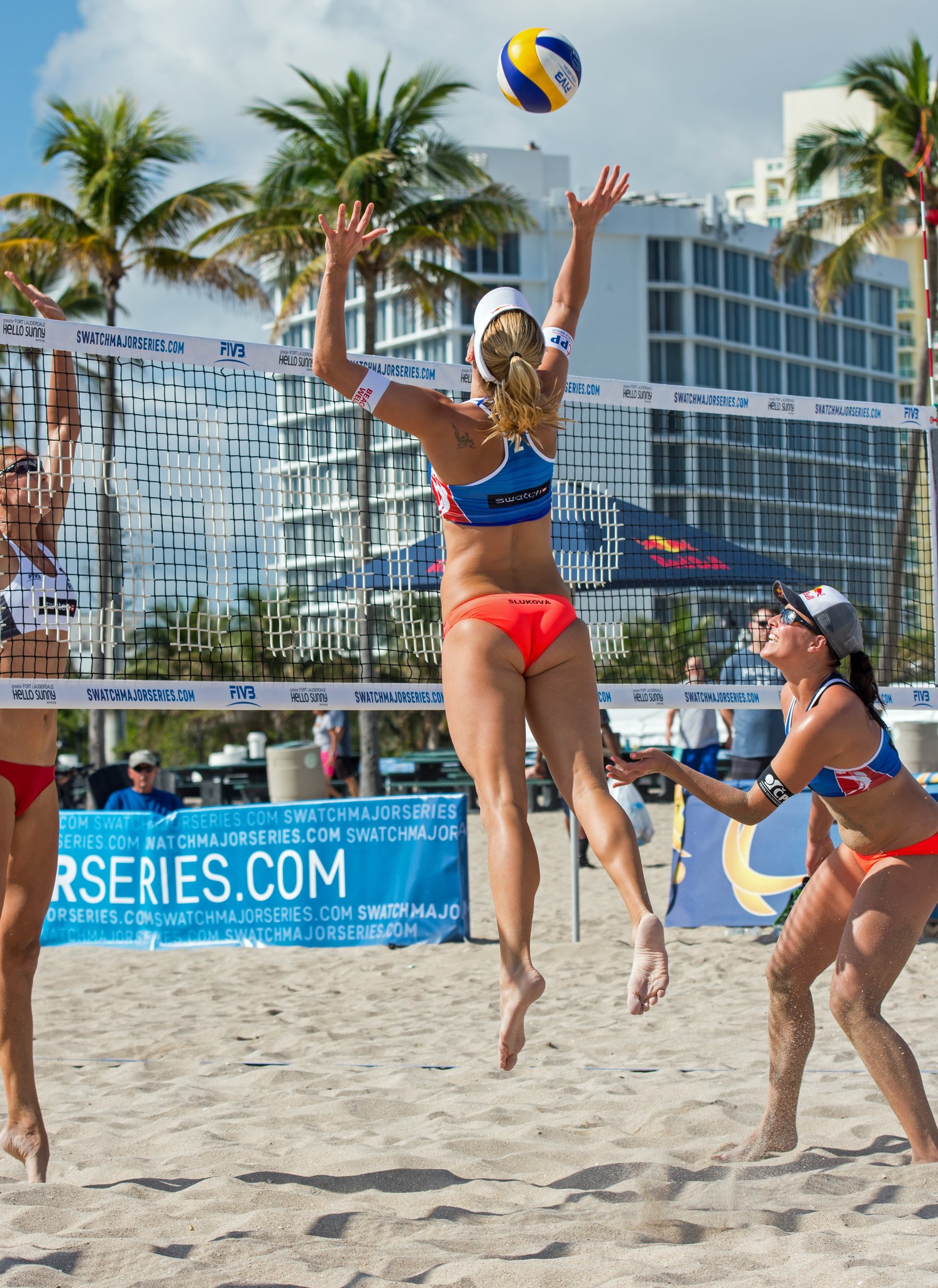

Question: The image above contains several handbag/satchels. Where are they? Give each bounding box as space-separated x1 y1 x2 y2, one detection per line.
607 765 655 847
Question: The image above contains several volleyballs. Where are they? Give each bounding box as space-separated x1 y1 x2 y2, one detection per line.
497 27 581 114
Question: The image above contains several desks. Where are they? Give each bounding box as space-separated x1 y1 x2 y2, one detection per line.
402 751 537 814
168 762 266 804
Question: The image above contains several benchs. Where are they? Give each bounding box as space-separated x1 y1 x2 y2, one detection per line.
392 781 550 788
177 780 250 788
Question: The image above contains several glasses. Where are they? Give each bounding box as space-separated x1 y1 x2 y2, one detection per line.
0 458 45 479
780 608 819 635
133 766 155 773
755 620 768 627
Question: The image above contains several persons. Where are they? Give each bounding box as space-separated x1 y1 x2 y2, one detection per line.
0 268 80 1192
718 607 787 780
665 656 732 781
102 749 186 817
606 580 938 1165
311 165 671 1073
309 710 360 799
532 710 620 868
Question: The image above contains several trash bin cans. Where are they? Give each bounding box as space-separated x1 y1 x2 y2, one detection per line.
267 740 325 805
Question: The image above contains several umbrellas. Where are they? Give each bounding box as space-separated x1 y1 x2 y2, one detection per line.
308 481 820 763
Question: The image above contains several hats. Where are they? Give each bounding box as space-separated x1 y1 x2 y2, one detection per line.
473 287 546 385
128 748 157 768
772 580 864 661
